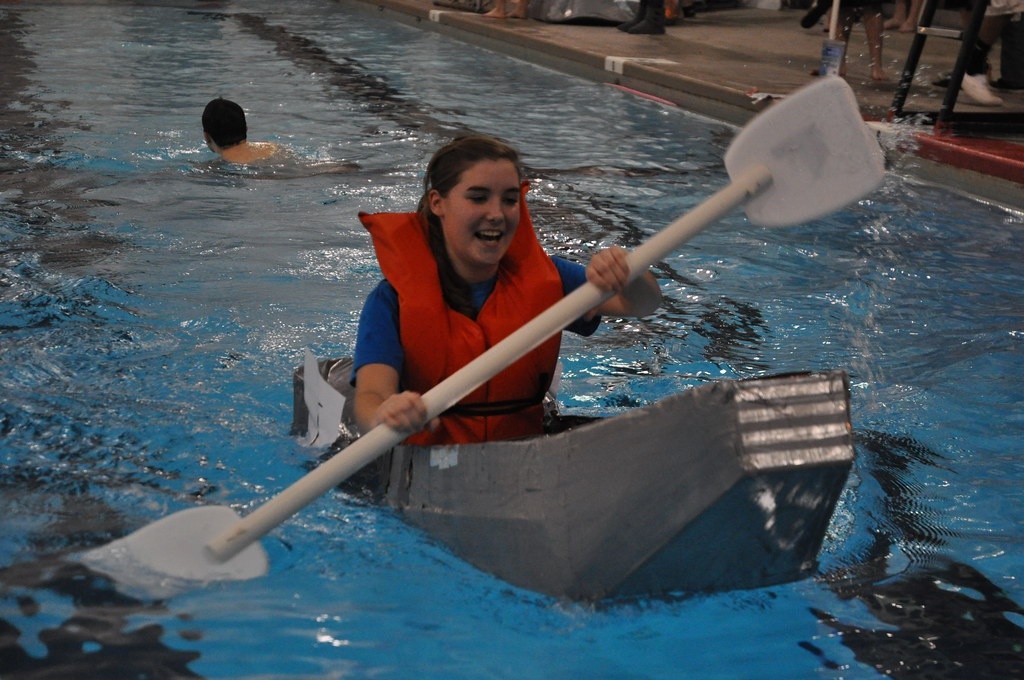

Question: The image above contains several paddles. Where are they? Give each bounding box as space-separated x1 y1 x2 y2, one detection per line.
75 75 886 591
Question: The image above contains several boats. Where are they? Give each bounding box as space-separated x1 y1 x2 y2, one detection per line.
291 357 854 614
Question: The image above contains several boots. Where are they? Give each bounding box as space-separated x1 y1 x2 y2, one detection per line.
628 0 664 34
618 0 649 32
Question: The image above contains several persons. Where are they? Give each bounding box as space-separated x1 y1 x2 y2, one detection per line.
616 0 696 36
348 132 663 450
483 0 530 19
808 0 1024 107
201 97 277 163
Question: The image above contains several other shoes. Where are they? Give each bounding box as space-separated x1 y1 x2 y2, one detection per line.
989 75 1024 93
800 0 833 28
961 73 1002 108
931 70 963 90
